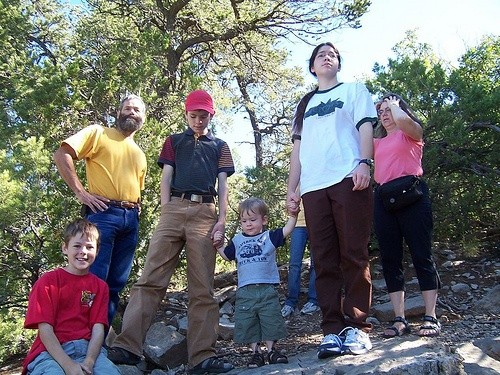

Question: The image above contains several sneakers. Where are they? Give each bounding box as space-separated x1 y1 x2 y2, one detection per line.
316 334 342 359
338 327 372 354
300 301 317 314
281 305 294 317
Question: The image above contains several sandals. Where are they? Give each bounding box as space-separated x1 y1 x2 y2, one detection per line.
247 350 265 369
418 316 441 337
190 358 234 373
382 317 411 338
267 348 288 364
108 347 141 365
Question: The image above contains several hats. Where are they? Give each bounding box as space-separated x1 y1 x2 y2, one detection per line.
186 90 214 114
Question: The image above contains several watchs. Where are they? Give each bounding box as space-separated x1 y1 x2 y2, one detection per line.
359 158 372 168
389 102 399 109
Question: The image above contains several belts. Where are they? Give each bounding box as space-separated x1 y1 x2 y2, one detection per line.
170 191 216 204
107 200 138 209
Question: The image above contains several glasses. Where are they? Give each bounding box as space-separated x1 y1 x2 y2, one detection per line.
378 108 391 115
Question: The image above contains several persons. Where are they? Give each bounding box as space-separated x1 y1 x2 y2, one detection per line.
212 197 300 368
286 42 378 358
281 181 321 319
372 92 441 338
54 94 146 347
21 219 123 375
106 90 235 373
